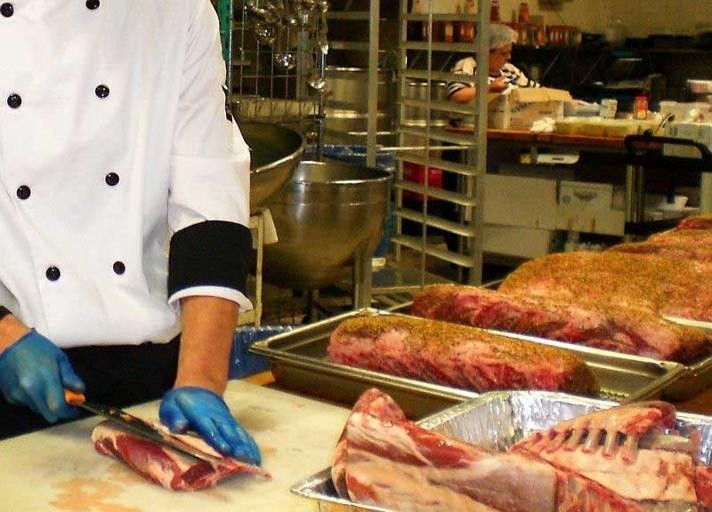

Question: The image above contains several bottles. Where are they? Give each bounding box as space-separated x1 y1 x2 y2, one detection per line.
413 0 582 49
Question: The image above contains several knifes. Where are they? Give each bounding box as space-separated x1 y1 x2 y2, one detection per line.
61 388 229 467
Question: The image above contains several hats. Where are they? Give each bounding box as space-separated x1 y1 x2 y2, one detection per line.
473 24 518 51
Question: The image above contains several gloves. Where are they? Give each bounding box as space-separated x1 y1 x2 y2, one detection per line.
159 387 262 464
0 328 86 422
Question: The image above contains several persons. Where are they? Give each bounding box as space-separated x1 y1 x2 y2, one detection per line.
447 25 542 128
1 0 261 463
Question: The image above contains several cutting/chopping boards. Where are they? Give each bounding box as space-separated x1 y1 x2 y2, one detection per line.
0 379 354 512
